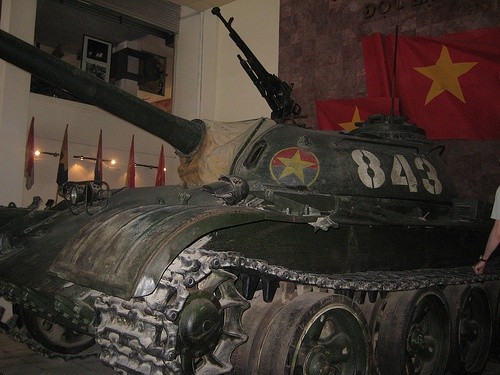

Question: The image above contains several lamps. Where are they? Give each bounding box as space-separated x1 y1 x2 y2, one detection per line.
201 175 250 207
61 181 113 215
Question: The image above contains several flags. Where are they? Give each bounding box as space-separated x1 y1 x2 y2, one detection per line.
25 118 35 191
94 128 103 187
315 96 398 135
124 133 136 191
362 29 500 140
57 124 69 196
152 144 166 189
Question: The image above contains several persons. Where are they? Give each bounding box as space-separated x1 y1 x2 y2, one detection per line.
43 199 54 211
474 185 500 275
28 196 42 209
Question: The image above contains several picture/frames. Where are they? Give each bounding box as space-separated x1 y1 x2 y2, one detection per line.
82 34 113 84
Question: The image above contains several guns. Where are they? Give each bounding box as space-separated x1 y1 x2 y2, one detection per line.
210 5 305 122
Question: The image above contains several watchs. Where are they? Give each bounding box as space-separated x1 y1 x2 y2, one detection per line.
479 255 487 263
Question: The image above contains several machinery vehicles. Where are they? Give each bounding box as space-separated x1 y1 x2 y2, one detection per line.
1 5 499 374
